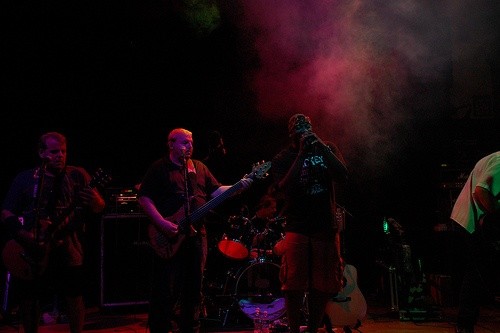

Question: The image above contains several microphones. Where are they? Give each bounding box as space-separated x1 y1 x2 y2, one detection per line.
45 156 52 162
180 145 187 161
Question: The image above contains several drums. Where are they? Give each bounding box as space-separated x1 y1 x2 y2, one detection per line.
217 214 258 259
249 232 275 258
234 260 287 320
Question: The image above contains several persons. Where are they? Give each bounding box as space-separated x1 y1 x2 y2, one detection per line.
135 128 253 333
0 131 106 333
269 113 348 333
449 151 500 333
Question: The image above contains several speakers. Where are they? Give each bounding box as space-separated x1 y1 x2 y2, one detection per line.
98 215 151 309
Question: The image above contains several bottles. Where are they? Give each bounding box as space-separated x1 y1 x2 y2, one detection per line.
253 310 262 333
261 310 269 333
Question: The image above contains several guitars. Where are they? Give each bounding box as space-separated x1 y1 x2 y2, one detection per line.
2 167 113 280
146 159 272 260
324 206 367 327
